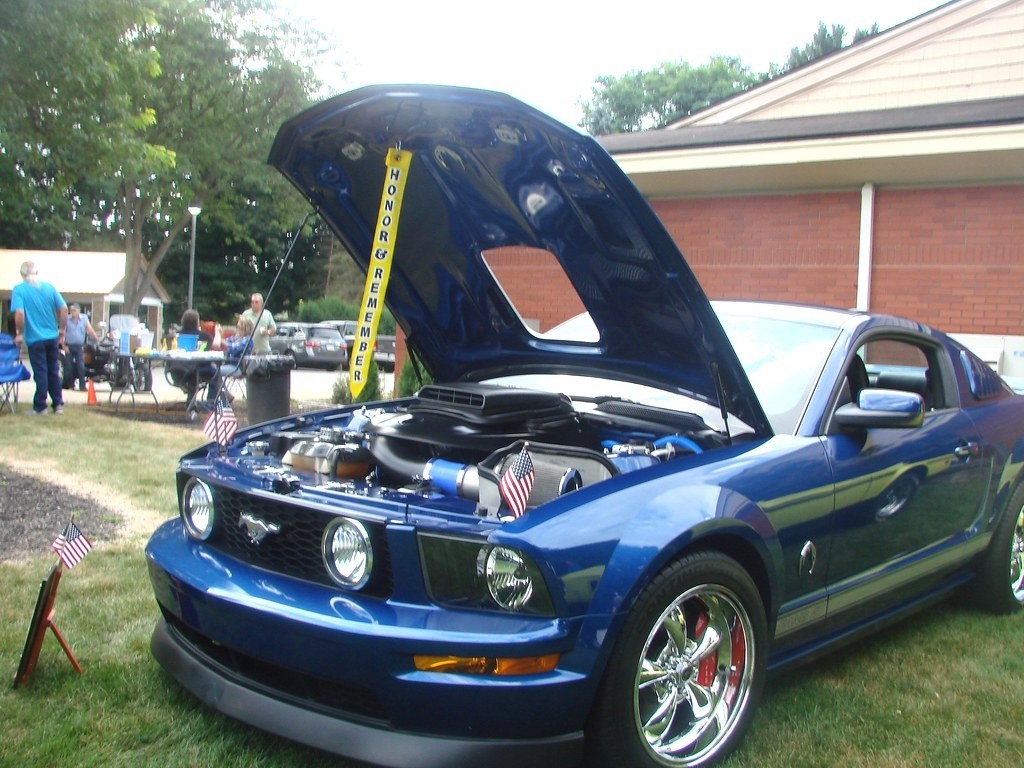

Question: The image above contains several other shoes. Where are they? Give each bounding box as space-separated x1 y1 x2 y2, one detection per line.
25 408 47 415
54 404 63 415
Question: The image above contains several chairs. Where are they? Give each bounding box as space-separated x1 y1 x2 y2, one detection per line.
0 333 30 415
876 371 929 406
846 353 871 404
163 333 227 409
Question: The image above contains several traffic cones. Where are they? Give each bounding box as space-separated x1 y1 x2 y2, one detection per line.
84 379 100 405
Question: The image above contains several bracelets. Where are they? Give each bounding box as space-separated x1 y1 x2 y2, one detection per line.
267 330 270 335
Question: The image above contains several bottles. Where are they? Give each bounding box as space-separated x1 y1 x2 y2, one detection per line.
120 329 129 354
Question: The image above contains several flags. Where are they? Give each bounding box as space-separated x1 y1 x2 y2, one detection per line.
500 448 535 520
202 391 238 445
52 522 92 569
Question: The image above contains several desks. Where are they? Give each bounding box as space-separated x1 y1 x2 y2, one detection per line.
109 350 229 423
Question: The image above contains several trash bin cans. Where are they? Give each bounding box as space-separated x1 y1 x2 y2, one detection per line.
242 356 296 426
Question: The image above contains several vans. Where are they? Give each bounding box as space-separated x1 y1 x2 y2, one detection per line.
270 323 347 372
145 82 1024 768
323 319 358 361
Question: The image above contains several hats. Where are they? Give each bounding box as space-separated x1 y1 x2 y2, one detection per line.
71 303 79 311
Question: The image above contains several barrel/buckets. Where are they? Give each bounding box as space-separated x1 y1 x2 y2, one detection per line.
176 333 199 351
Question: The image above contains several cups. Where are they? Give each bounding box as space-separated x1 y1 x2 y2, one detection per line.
140 333 153 350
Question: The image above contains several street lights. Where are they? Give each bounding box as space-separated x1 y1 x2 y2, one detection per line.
187 207 201 310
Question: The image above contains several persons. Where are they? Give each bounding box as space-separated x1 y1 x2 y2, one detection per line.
213 317 254 407
62 302 100 391
11 262 68 415
170 309 225 414
162 327 177 350
241 293 277 355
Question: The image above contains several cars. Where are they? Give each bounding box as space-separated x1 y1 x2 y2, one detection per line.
372 334 397 371
